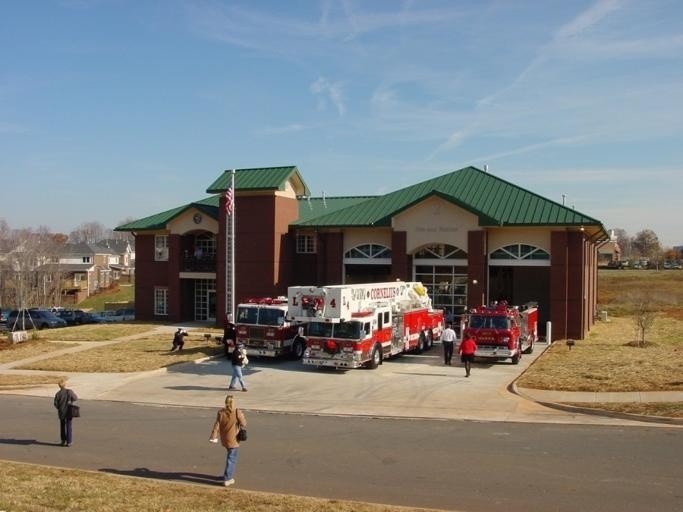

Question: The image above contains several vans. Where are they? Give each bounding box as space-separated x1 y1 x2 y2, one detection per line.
7 309 68 330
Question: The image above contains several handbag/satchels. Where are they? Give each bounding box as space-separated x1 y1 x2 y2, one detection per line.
238 429 246 441
67 405 80 417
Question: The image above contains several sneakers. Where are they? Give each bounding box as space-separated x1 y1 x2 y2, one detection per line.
223 479 235 487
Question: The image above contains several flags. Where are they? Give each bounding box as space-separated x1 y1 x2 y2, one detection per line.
224 186 233 217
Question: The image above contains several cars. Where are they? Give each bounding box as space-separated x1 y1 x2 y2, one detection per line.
51 307 135 326
608 258 682 270
0 310 11 323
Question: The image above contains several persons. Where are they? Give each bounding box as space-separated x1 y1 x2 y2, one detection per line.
440 324 456 365
458 334 477 377
170 328 188 353
194 246 203 260
208 395 246 486
54 378 77 447
229 343 249 392
223 322 235 355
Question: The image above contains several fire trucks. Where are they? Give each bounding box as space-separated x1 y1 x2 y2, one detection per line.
286 278 446 370
464 299 539 365
235 295 306 362
